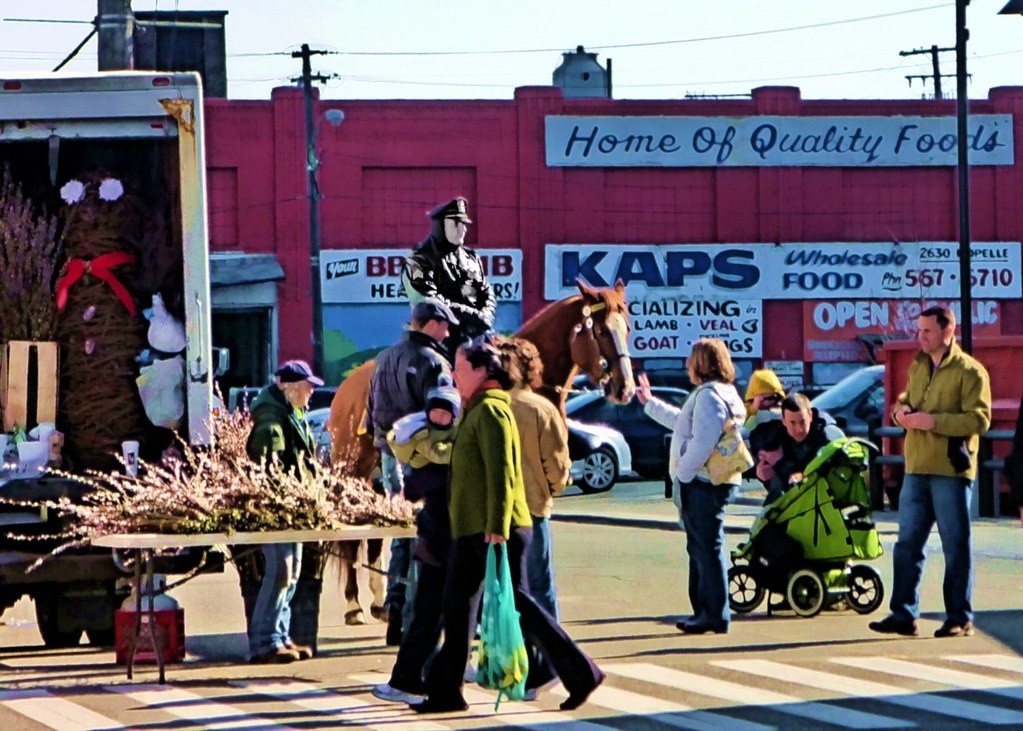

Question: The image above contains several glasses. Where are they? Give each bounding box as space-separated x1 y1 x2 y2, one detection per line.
761 393 778 403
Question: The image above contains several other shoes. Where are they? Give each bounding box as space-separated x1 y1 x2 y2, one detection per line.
408 696 468 715
523 677 561 700
463 663 476 683
560 674 605 711
934 617 974 638
869 612 919 636
251 644 300 664
284 642 313 660
678 617 729 635
387 631 405 647
373 684 427 704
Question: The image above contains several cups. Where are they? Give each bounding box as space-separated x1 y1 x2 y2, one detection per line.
122 441 139 477
0 435 8 472
39 423 55 459
17 442 48 478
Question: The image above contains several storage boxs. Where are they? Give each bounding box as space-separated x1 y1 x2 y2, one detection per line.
114 607 185 664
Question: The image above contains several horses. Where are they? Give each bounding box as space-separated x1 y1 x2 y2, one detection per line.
327 277 637 626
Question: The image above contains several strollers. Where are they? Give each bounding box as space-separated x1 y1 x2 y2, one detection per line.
727 437 884 617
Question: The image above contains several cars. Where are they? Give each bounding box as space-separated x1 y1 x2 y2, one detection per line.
299 363 884 498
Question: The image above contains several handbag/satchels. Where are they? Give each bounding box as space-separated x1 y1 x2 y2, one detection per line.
694 387 755 485
476 537 528 701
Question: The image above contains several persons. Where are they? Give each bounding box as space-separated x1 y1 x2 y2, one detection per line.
410 343 605 714
635 338 744 636
401 194 497 359
869 306 992 638
742 368 851 611
366 298 606 705
1005 394 1023 531
247 360 325 663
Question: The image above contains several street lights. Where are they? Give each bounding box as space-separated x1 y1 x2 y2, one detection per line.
305 80 344 388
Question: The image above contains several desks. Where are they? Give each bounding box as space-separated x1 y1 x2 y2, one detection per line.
91 524 417 683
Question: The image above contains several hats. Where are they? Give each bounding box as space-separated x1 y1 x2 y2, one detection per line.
426 385 461 429
412 297 460 325
273 360 325 386
429 196 472 224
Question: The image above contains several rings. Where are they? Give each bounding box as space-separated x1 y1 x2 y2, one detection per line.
913 421 916 425
